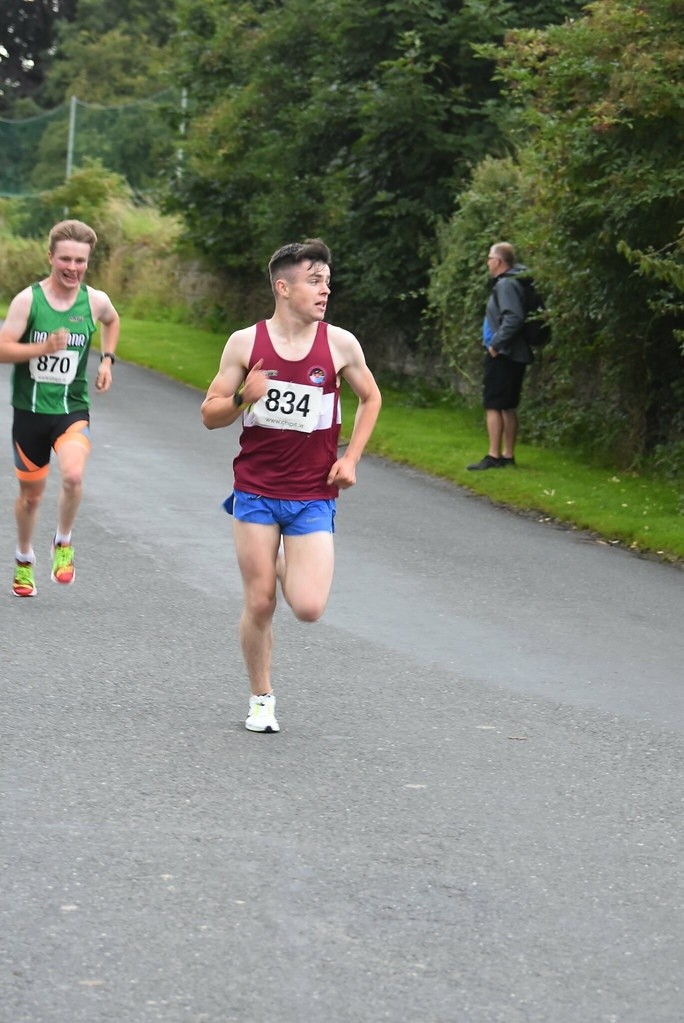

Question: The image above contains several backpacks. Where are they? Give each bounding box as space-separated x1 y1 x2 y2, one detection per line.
489 273 552 348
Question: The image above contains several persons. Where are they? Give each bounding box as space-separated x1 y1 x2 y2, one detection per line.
201 237 383 732
467 242 535 471
2 220 118 597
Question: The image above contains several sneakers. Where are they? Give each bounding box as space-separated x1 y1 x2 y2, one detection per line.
467 454 515 472
11 558 38 598
278 534 285 557
245 688 280 734
50 536 76 587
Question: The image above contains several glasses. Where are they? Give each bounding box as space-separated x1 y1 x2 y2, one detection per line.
487 256 498 262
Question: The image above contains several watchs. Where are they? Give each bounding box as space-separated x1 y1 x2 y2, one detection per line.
99 351 116 365
233 391 244 408
